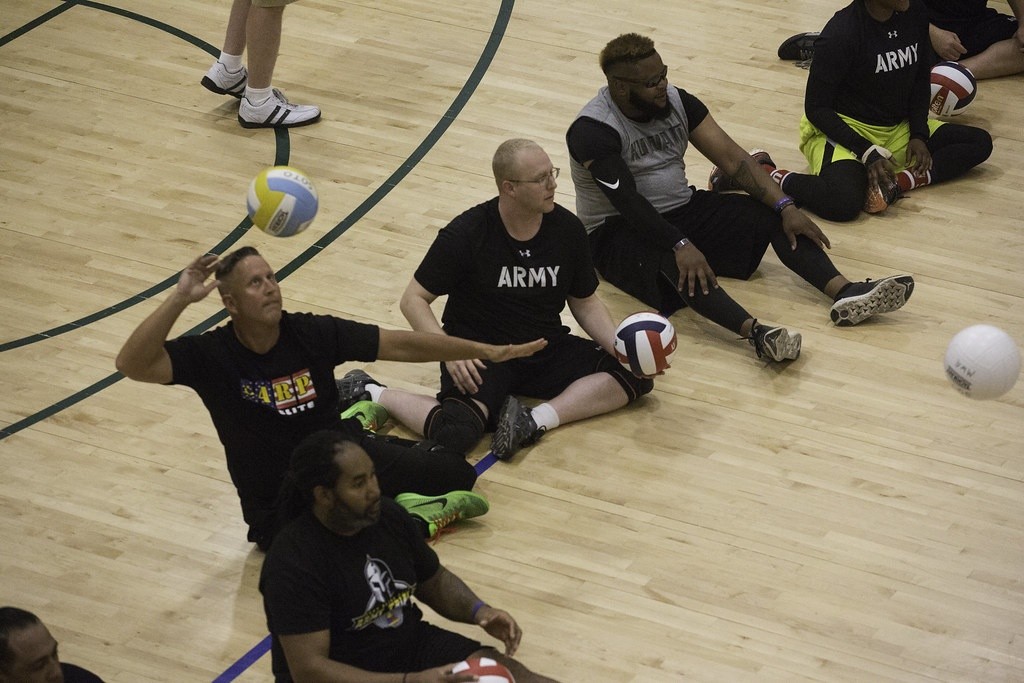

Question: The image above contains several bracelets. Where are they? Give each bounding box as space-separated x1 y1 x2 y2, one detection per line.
672 238 689 253
769 194 795 213
471 601 484 623
402 673 408 683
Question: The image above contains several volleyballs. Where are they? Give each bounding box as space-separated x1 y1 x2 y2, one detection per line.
943 323 1022 402
454 656 516 683
612 311 678 376
928 59 978 115
245 163 320 238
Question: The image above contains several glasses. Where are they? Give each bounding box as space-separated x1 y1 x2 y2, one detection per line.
507 167 560 187
613 65 667 88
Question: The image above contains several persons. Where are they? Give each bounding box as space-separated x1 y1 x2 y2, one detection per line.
201 0 321 129
565 33 915 363
115 246 548 553
0 606 105 683
778 0 1024 79
258 428 559 683
334 138 655 460
709 0 993 223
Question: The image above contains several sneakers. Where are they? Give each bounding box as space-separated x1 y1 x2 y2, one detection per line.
395 490 490 545
863 176 911 213
830 274 914 327
778 32 821 69
340 400 389 434
490 395 546 459
708 148 777 191
201 60 321 128
736 318 802 362
334 369 387 413
